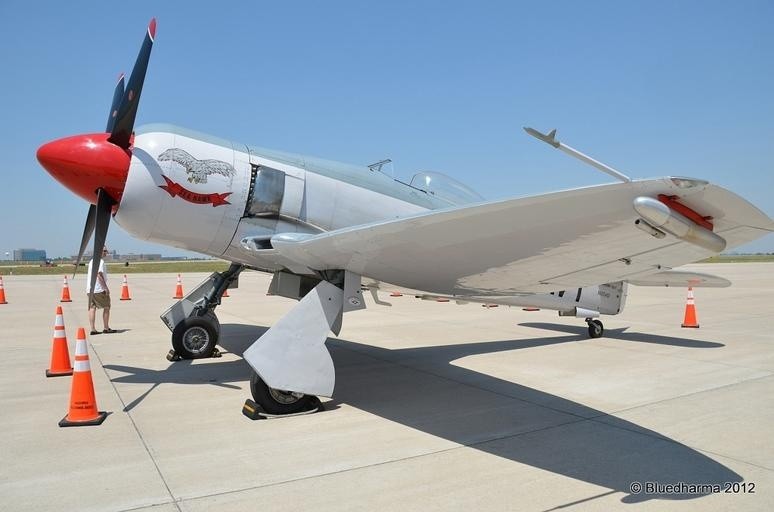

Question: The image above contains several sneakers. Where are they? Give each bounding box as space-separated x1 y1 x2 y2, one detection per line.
90 331 103 335
103 328 117 333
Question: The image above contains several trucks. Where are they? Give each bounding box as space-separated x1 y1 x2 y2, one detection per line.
39 260 58 267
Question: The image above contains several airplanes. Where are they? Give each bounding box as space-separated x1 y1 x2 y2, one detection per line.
35 14 774 424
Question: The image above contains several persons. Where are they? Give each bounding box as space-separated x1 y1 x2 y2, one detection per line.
85 245 115 334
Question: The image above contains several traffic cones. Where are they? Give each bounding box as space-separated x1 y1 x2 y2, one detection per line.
0 277 9 305
43 305 76 378
61 275 73 304
57 326 110 426
390 290 541 313
221 286 231 298
173 273 186 300
679 284 705 332
118 273 134 301
